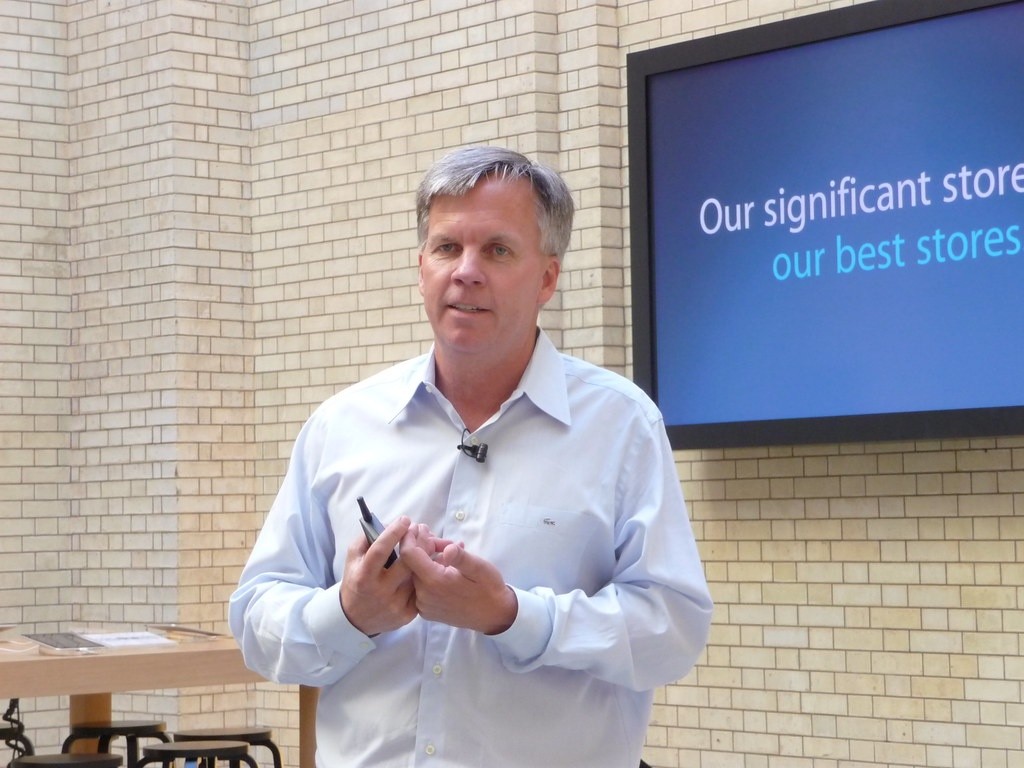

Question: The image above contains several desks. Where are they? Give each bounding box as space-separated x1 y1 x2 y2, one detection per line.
0 626 319 768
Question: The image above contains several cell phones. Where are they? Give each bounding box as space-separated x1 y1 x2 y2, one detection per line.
356 496 398 569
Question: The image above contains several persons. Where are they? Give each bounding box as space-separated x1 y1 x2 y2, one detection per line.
228 147 713 768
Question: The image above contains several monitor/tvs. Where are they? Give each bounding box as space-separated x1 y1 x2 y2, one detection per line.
627 1 1023 452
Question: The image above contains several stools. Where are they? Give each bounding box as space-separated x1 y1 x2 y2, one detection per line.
0 724 35 755
61 720 173 768
174 727 282 768
7 753 123 768
134 740 258 768
